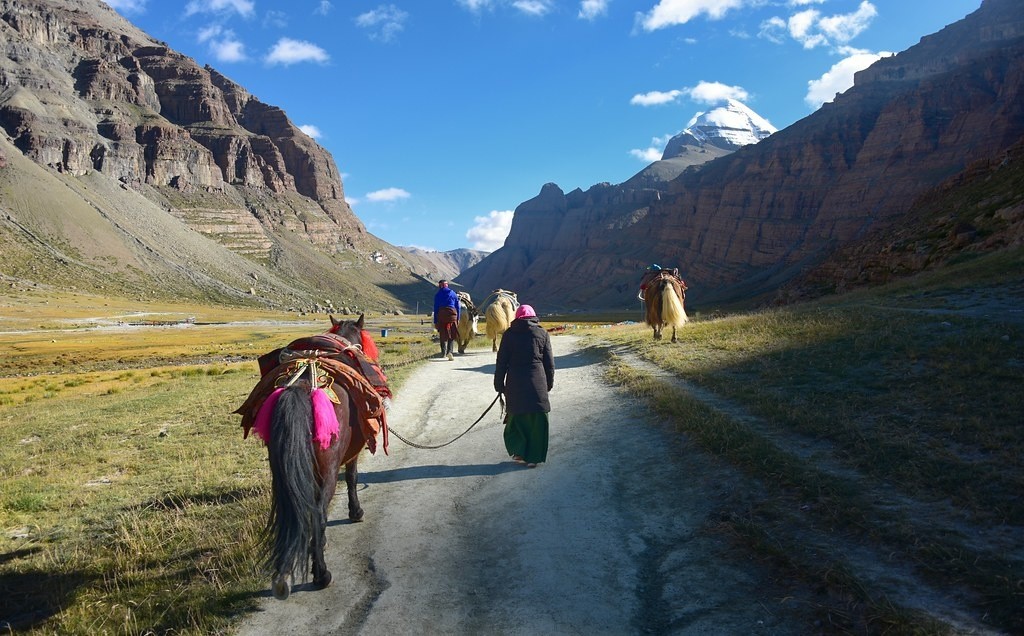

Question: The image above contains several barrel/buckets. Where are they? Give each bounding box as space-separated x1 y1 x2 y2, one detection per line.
382 330 388 337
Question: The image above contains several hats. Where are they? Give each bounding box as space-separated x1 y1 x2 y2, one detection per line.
516 305 536 318
439 280 449 287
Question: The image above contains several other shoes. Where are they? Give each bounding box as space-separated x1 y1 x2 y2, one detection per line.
528 462 536 467
443 355 447 358
447 353 454 361
513 454 521 460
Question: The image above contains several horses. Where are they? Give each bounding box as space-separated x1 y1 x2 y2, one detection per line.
486 288 518 352
250 313 365 600
647 274 689 344
454 292 479 354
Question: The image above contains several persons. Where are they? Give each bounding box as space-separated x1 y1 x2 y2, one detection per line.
493 304 555 467
433 280 461 362
